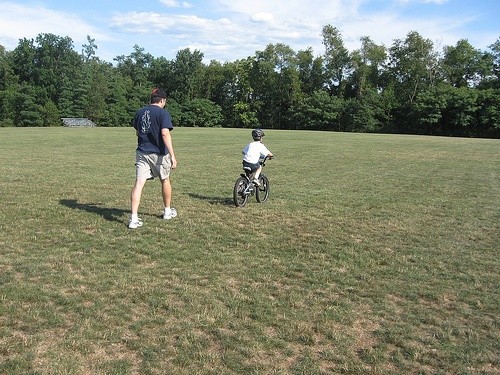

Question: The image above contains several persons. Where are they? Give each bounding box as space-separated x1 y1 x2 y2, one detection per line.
242 129 273 197
129 90 178 229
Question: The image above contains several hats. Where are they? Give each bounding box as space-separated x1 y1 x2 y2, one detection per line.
151 89 167 98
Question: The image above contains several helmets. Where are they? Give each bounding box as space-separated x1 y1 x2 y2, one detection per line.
252 129 265 137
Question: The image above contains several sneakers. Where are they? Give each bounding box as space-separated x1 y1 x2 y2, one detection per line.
163 208 177 220
129 218 143 229
252 179 261 187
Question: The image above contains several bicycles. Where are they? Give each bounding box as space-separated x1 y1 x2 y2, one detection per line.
233 155 274 207
